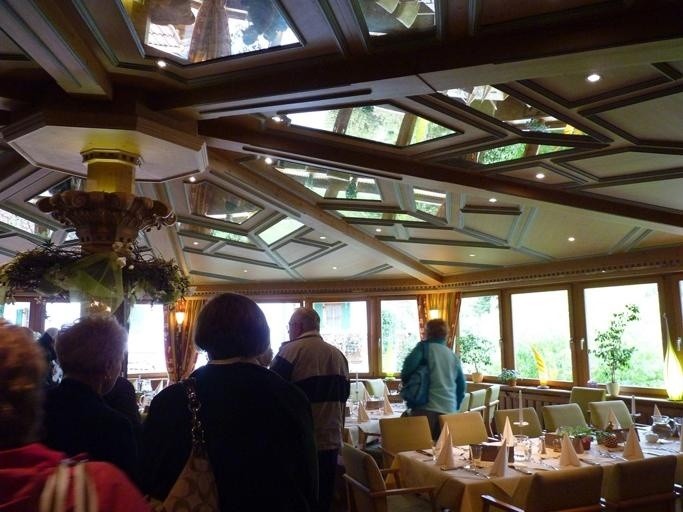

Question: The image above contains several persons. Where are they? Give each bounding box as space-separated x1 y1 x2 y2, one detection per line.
270 308 349 512
400 319 467 441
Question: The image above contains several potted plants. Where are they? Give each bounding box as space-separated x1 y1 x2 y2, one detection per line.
455 335 496 383
587 303 639 396
498 367 521 387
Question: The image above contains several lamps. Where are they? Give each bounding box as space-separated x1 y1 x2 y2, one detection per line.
174 310 184 333
429 307 440 319
529 343 549 390
660 314 681 401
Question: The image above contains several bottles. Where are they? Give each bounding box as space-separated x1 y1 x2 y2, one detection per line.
538 429 547 456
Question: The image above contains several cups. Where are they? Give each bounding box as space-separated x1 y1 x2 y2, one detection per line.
650 415 669 424
513 433 528 461
552 438 561 452
470 446 481 463
581 436 590 449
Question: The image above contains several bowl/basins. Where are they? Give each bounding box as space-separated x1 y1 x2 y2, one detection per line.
645 435 658 442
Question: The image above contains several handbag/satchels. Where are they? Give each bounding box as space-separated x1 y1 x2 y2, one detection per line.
400 340 430 409
156 377 222 511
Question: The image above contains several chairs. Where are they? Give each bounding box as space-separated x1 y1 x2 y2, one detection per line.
337 378 682 512
